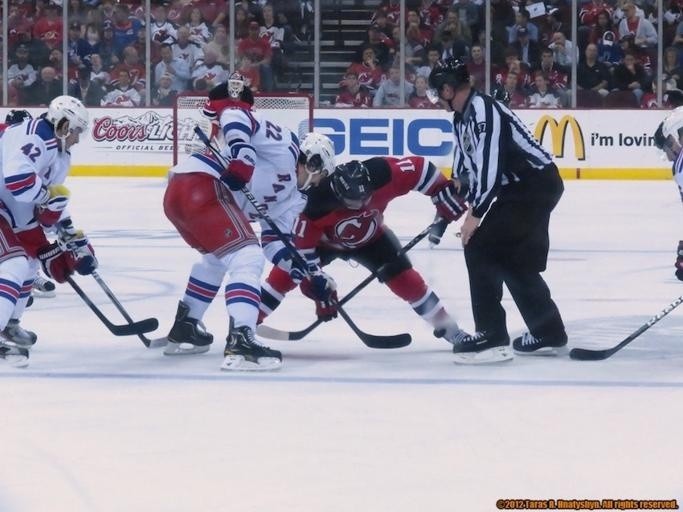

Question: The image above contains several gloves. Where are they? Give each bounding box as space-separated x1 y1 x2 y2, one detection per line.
57 231 97 274
430 180 468 222
37 240 76 283
40 186 69 212
220 143 257 191
289 262 337 322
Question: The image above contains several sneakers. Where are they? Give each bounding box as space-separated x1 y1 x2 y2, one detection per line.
437 323 567 353
675 241 683 282
168 301 213 346
1 319 37 346
224 315 281 363
0 342 28 356
33 273 55 291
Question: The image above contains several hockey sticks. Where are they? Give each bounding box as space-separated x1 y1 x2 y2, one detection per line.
255 215 449 342
62 272 160 337
191 124 412 350
567 293 683 363
91 268 175 350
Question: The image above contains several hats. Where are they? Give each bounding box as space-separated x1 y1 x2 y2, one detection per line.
547 8 561 16
603 31 616 47
517 27 527 33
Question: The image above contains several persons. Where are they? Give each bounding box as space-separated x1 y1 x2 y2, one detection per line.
0 0 682 109
257 156 472 345
1 109 55 311
202 70 255 145
429 56 569 352
0 96 99 364
163 108 339 363
652 108 683 282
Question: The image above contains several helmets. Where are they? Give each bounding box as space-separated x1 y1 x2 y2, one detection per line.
425 57 470 104
46 96 88 131
654 105 683 161
297 133 336 192
6 110 34 126
227 72 245 98
330 160 371 210
